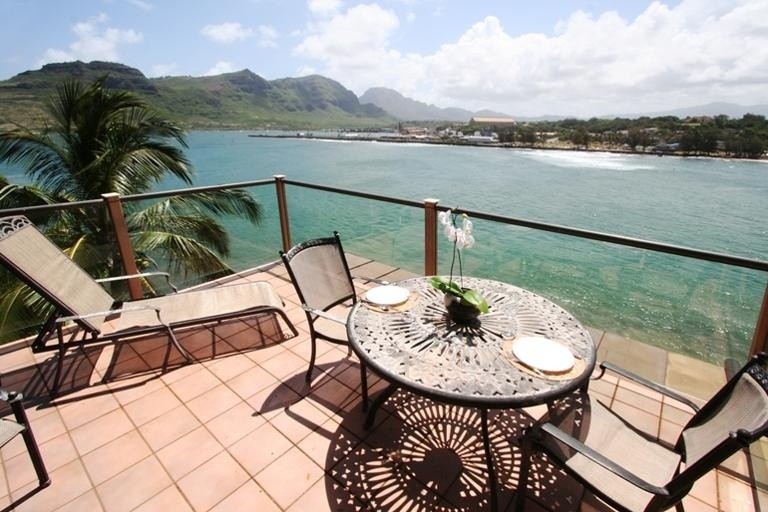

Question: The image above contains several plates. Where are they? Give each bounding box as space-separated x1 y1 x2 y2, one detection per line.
512 334 575 373
366 284 411 306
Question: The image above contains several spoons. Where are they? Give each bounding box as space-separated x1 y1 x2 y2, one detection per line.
360 299 390 313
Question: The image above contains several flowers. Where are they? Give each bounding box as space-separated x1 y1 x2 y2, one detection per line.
431 204 488 318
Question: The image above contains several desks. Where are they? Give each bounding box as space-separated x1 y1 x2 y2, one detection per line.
345 272 601 503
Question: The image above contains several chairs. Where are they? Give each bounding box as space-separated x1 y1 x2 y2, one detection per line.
0 214 298 397
279 233 396 427
518 349 765 510
